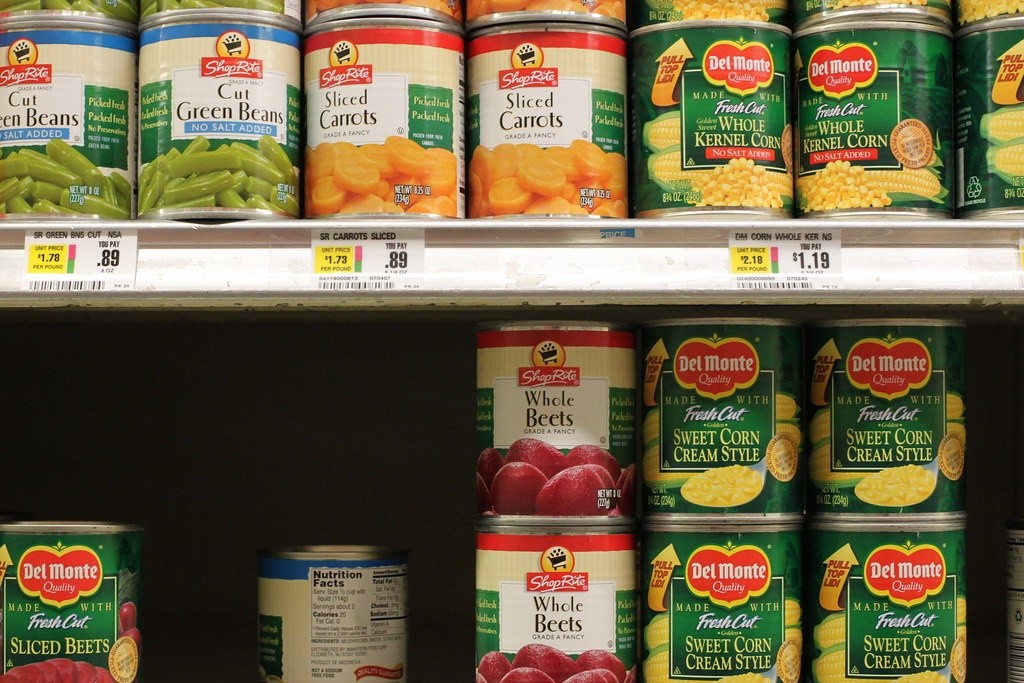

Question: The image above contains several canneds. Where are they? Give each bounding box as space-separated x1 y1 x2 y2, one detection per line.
0 0 1024 683
